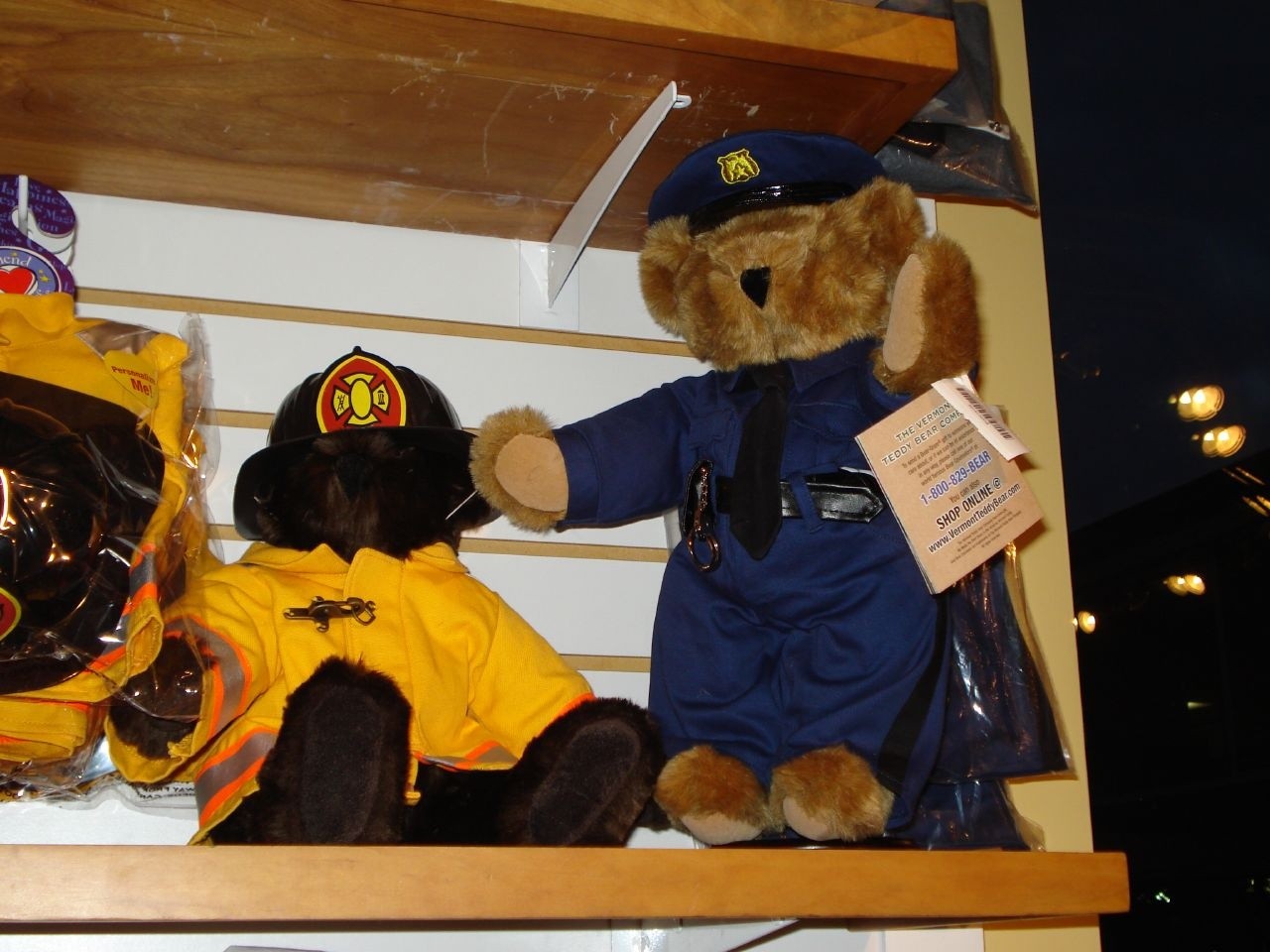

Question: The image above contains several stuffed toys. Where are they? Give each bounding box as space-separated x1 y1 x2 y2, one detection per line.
110 127 988 848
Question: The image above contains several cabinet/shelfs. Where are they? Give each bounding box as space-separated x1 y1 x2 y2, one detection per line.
0 0 1131 952
